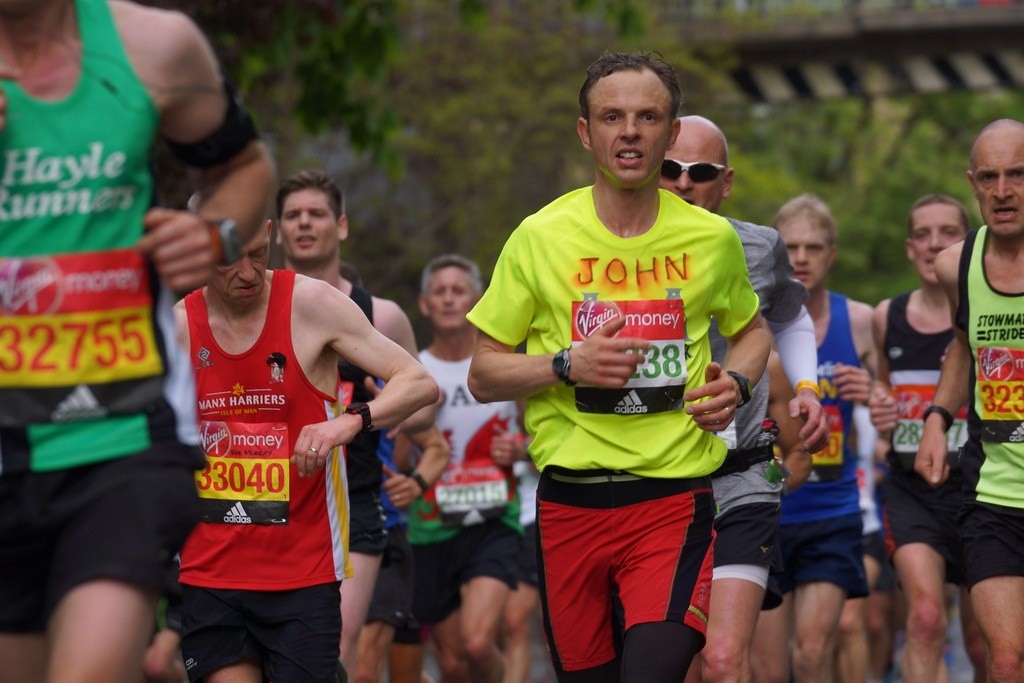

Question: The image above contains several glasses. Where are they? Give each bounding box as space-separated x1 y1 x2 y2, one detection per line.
661 159 726 183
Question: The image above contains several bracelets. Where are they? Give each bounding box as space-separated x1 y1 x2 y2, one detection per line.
411 471 430 491
923 405 954 433
793 380 821 399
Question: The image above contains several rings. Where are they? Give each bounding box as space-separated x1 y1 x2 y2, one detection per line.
309 447 318 453
725 407 731 416
395 494 402 500
495 449 503 458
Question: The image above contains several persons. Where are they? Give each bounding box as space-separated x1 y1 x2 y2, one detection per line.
659 114 829 683
172 192 439 683
275 168 422 683
913 118 1024 683
144 192 974 683
0 0 276 683
466 49 773 683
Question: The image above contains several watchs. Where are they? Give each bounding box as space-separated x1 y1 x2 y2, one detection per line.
345 402 374 438
552 349 576 387
208 217 240 265
726 371 752 409
